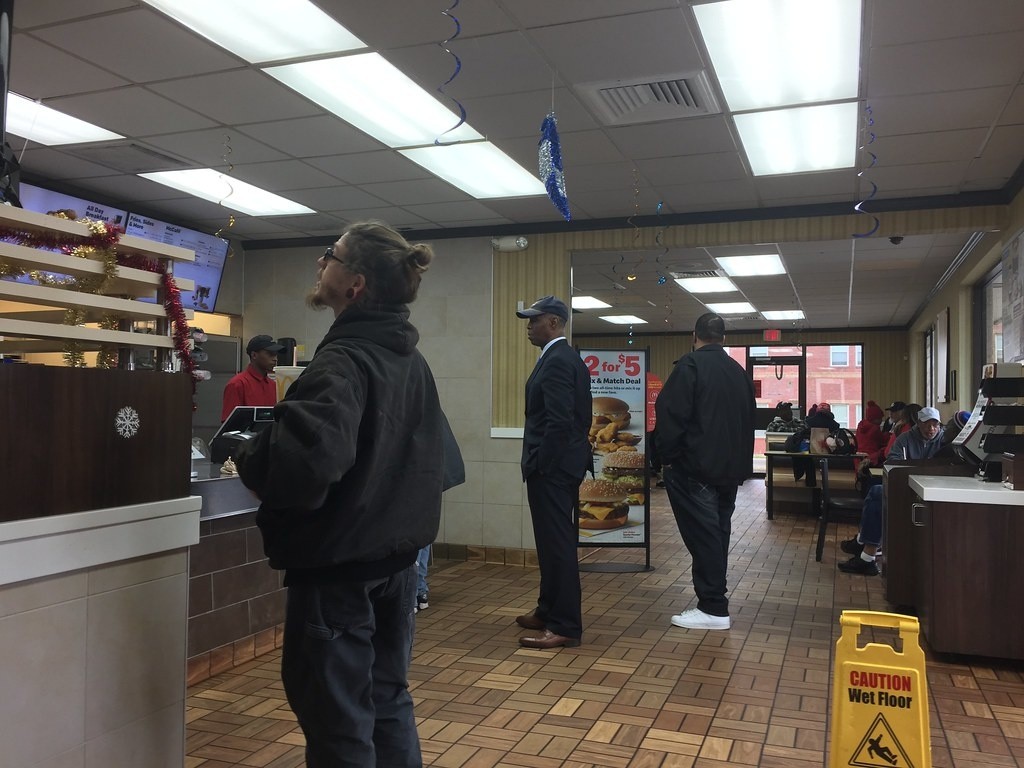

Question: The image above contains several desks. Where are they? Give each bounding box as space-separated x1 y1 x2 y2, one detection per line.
764 432 856 484
762 450 869 521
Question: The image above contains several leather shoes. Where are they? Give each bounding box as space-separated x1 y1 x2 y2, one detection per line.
519 627 581 649
515 615 545 629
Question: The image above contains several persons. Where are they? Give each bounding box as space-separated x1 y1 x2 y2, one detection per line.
516 296 596 649
413 408 465 614
230 222 444 768
221 335 287 424
838 400 971 576
655 314 756 629
767 400 851 455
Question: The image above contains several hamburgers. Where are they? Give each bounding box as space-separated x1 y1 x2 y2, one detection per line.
602 452 646 506
578 480 630 528
590 397 631 429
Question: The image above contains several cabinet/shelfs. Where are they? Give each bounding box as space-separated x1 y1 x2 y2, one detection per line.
910 488 1024 663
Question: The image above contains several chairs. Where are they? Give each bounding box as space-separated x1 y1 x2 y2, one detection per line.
815 458 866 560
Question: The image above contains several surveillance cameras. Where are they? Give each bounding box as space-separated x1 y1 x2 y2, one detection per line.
889 236 905 245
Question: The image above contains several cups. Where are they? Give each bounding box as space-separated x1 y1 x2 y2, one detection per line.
191 353 207 362
192 370 211 380
273 365 306 403
193 332 208 342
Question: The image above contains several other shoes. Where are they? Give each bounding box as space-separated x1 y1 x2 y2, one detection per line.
876 544 883 556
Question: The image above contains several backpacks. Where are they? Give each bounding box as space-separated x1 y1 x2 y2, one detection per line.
825 428 858 454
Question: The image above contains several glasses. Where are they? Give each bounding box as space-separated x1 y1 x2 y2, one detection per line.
323 247 370 291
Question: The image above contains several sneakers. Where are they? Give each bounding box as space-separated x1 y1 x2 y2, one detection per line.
656 481 666 489
841 533 865 555
838 557 878 576
413 593 429 613
670 609 731 630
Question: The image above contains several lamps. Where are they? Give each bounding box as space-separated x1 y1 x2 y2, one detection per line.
489 234 529 252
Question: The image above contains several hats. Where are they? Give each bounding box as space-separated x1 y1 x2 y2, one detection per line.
816 403 831 414
953 410 971 429
776 401 792 415
246 335 285 355
865 400 884 421
919 406 941 423
885 401 906 412
515 296 569 323
808 404 819 417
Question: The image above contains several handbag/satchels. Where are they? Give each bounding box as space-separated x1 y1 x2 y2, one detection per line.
810 428 829 454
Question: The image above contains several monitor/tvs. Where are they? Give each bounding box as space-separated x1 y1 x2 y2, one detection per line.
209 406 274 447
